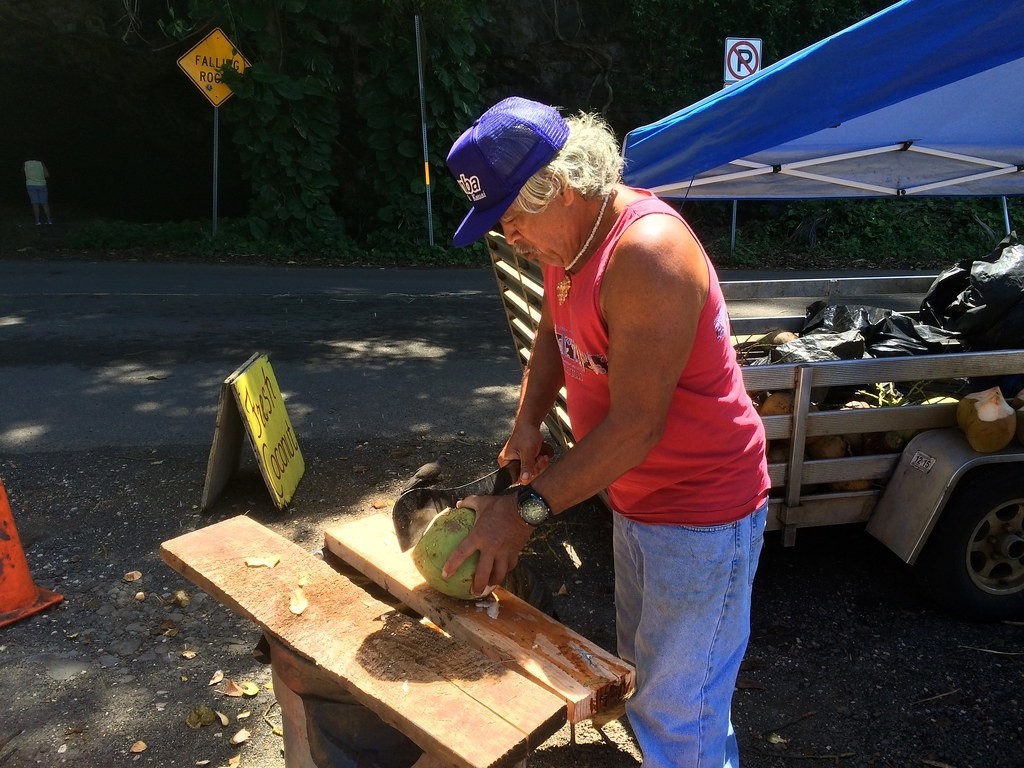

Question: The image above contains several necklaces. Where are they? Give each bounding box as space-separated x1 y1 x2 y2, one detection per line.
557 189 610 308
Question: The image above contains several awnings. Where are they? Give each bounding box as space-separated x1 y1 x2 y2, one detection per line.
620 2 1023 197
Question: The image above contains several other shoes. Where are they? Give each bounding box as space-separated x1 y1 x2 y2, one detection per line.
34 219 41 225
44 218 55 224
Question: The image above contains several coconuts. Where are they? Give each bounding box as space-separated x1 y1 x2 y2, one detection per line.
411 507 497 600
752 328 959 491
1012 389 1024 447
955 386 1016 453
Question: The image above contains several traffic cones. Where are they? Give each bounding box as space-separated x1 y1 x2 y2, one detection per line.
0 476 63 630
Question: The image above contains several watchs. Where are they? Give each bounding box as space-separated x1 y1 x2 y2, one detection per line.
518 485 551 526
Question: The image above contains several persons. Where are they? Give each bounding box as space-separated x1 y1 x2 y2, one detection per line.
440 97 771 768
18 149 53 226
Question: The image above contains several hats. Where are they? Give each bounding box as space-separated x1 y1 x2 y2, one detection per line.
452 96 568 251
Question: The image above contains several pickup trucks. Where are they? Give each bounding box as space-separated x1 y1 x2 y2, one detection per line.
623 0 1024 605
